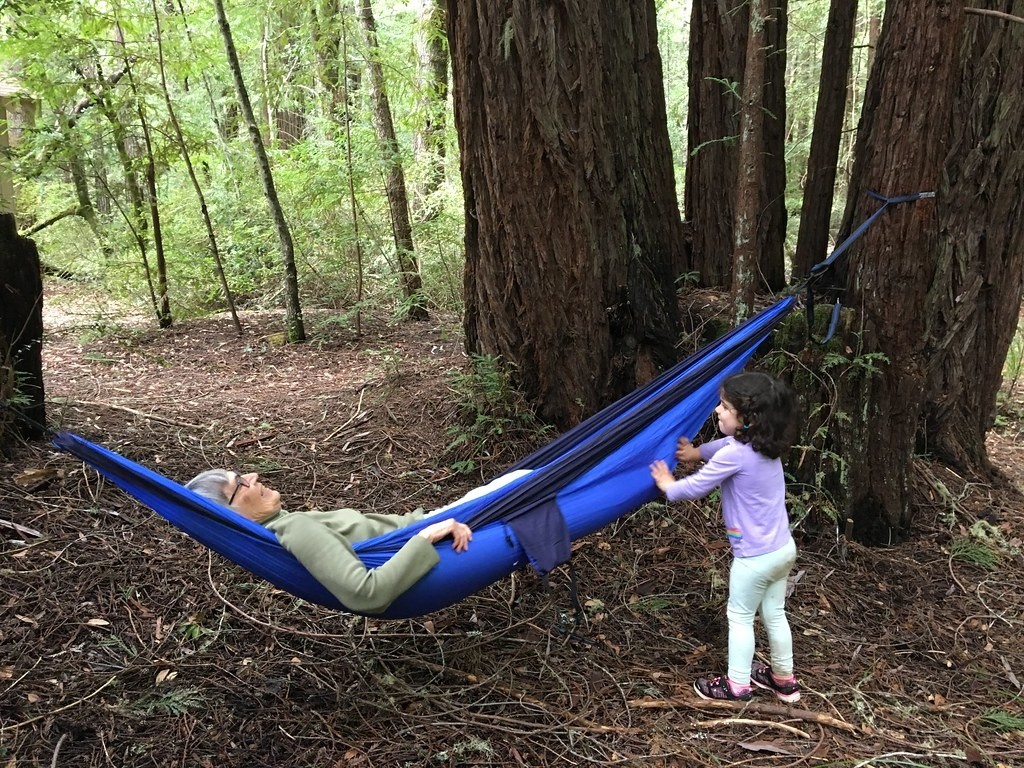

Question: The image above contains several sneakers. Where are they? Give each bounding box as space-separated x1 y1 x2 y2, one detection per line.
751 662 801 704
693 675 752 703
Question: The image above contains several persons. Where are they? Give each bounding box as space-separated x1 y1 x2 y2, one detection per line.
648 372 801 704
183 469 536 615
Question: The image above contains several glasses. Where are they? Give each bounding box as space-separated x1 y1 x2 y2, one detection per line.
229 473 250 505
